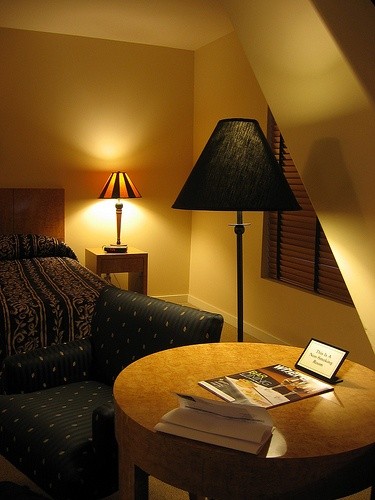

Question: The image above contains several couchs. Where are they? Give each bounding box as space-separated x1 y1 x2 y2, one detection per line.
0 286 224 500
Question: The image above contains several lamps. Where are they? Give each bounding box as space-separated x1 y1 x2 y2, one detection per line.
171 118 302 342
98 171 143 246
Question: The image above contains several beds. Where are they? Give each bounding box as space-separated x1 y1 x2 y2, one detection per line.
0 232 116 381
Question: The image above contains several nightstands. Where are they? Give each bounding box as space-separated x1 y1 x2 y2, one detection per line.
85 247 148 296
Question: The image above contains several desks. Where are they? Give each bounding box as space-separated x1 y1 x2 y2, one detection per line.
113 342 375 500
